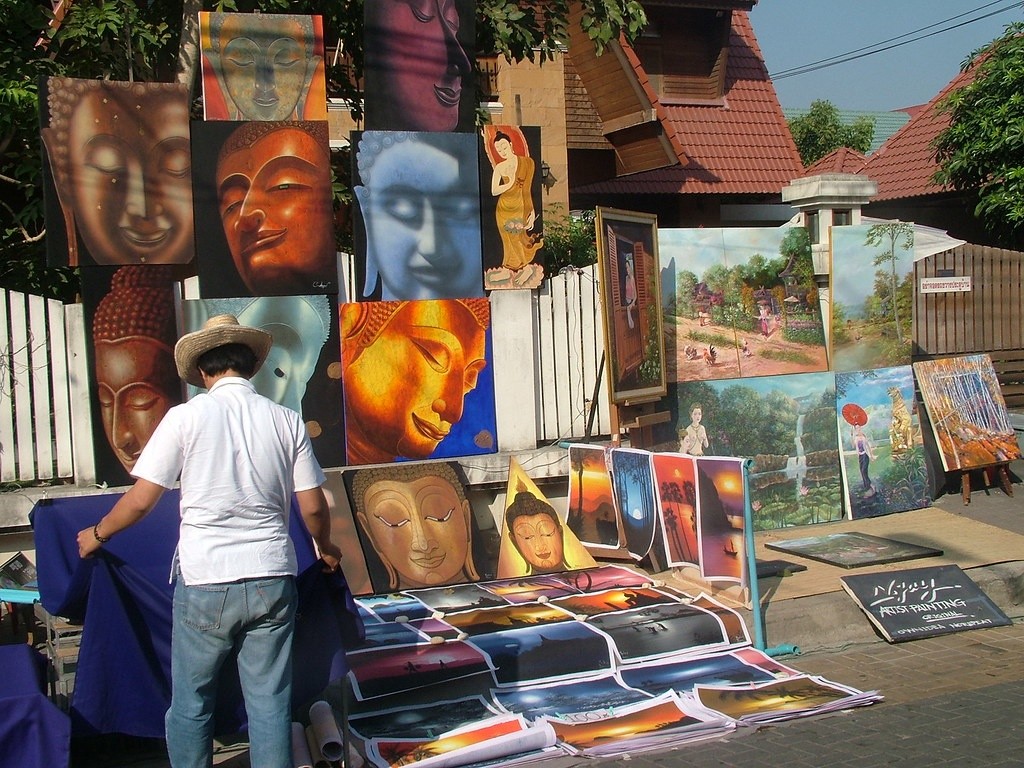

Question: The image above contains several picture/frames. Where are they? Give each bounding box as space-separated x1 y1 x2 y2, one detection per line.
593 205 667 405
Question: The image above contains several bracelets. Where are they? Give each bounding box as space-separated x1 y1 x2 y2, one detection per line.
94 523 111 543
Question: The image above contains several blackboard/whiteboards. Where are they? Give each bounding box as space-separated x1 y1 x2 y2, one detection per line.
764 531 944 571
840 564 1013 643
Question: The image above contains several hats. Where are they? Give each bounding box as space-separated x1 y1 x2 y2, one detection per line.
173 313 272 389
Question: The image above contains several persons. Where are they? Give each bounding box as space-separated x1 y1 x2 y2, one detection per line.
77 315 343 768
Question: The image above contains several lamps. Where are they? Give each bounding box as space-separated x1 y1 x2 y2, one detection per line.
537 159 551 185
